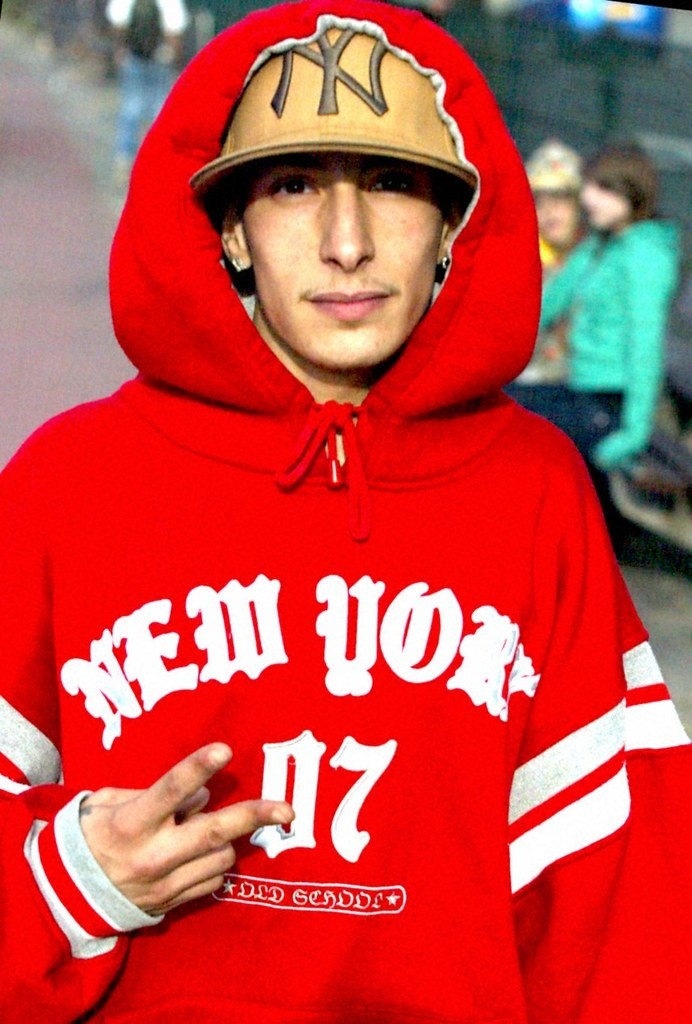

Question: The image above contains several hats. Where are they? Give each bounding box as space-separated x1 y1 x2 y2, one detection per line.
190 25 477 190
524 140 585 191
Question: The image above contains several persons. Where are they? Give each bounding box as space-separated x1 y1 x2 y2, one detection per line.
0 0 692 1024
103 0 195 193
504 136 686 568
